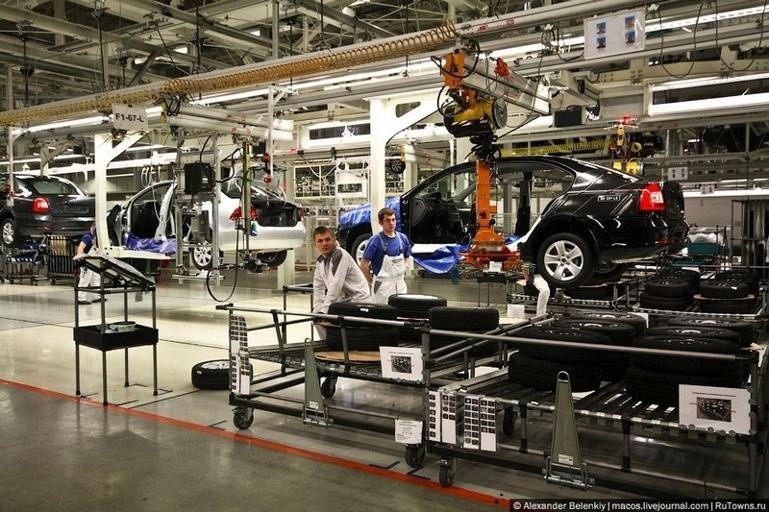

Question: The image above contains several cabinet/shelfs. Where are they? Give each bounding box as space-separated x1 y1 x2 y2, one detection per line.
216 299 769 512
71 251 161 405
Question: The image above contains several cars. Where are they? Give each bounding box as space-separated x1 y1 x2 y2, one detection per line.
1 173 96 248
89 178 306 270
335 155 690 288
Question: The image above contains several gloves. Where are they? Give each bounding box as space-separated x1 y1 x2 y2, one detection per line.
313 312 325 322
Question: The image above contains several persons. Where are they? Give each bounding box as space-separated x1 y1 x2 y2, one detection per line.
519 260 551 317
360 208 414 306
74 221 108 305
309 225 372 341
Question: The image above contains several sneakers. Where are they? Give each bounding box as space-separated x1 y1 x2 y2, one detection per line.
78 298 107 305
